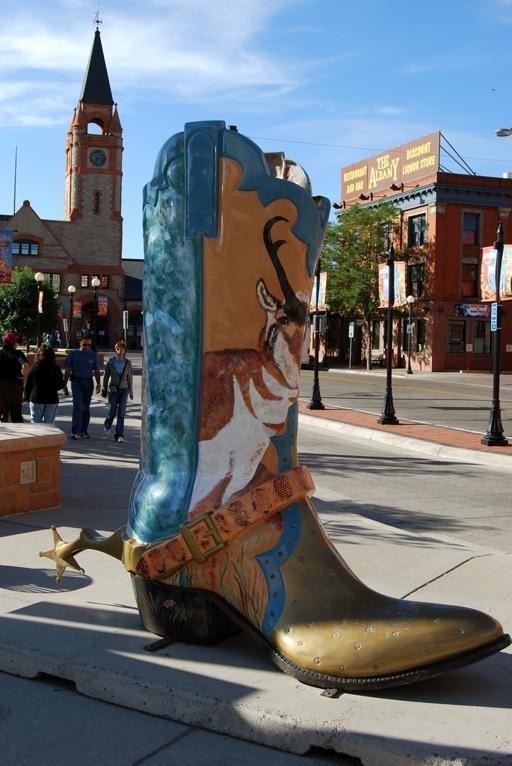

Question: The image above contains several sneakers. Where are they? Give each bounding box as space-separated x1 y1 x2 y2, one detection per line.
117 436 124 443
71 432 90 440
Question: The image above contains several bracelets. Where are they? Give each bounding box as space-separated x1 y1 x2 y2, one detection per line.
97 383 100 386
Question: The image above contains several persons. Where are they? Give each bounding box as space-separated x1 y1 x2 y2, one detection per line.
101 338 134 442
24 345 65 423
0 332 30 423
63 333 100 440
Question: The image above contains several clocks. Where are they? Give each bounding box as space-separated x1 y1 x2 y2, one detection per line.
90 148 109 167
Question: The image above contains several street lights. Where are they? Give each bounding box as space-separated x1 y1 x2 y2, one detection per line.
68 285 78 348
405 295 414 373
89 275 102 353
35 272 45 348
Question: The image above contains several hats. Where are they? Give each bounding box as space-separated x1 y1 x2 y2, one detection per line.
3 334 20 343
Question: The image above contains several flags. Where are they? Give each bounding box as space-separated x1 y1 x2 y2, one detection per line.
0 229 15 284
478 246 497 302
376 263 389 309
317 271 328 312
38 291 44 314
498 244 512 301
308 275 317 313
392 260 407 308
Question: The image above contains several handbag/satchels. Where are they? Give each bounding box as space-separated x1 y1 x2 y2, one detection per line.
110 384 118 393
